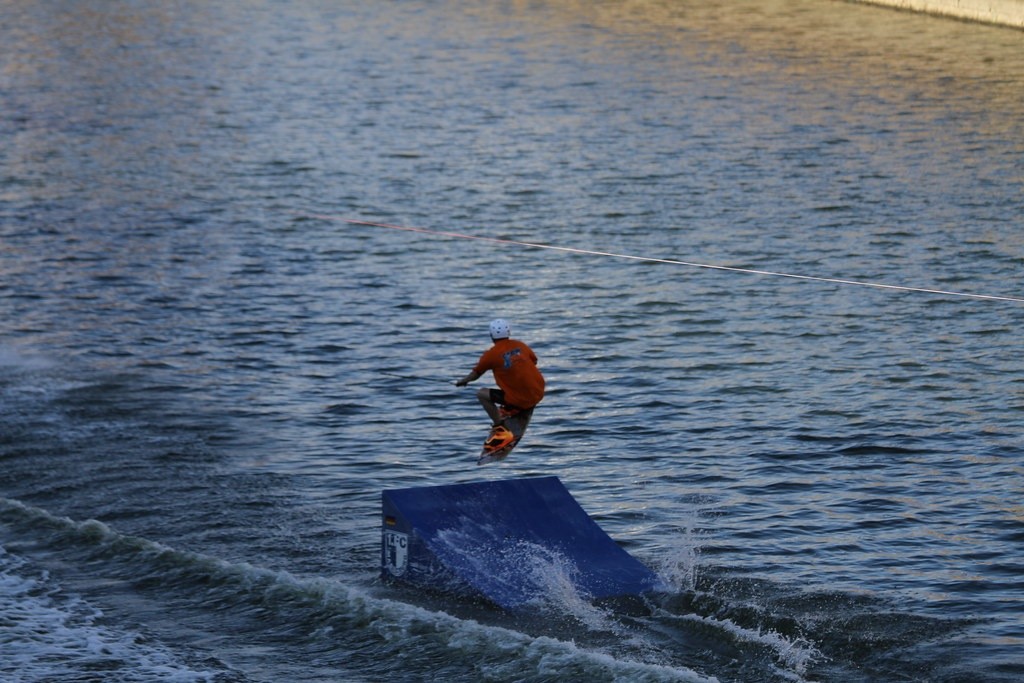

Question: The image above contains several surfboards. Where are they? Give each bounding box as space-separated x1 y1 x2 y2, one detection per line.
478 404 536 466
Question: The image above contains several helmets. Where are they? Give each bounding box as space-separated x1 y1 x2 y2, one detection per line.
490 318 510 339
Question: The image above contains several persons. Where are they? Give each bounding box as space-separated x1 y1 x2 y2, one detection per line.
456 320 545 454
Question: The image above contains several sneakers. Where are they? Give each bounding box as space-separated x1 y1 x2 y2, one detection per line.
496 405 520 416
484 420 510 450
